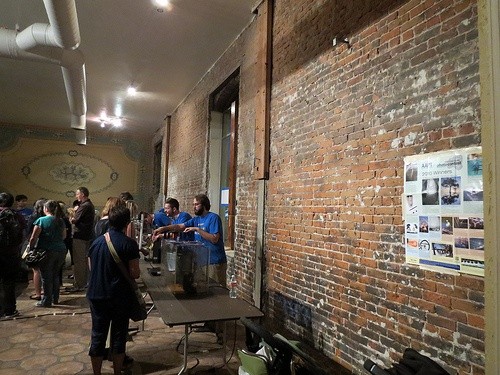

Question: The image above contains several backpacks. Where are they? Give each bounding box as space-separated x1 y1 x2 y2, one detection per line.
0 207 24 265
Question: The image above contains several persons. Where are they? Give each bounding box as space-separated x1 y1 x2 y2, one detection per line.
153 193 227 345
0 191 28 318
138 211 172 230
94 191 138 369
87 205 140 375
14 194 82 301
152 197 195 242
65 187 95 292
26 200 67 307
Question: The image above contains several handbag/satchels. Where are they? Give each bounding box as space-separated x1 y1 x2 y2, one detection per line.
110 269 147 322
25 249 49 268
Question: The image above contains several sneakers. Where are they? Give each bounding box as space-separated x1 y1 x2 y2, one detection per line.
0 310 23 320
52 299 58 304
65 286 85 291
34 301 51 307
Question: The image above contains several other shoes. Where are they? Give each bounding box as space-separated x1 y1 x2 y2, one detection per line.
218 336 223 344
29 295 41 300
68 275 74 279
108 347 133 364
193 325 214 332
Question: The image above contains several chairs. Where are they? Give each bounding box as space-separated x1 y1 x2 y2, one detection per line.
235 345 276 375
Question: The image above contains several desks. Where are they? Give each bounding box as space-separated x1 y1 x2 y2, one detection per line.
139 248 264 375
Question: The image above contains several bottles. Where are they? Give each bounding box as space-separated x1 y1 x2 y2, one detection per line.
230 275 237 298
